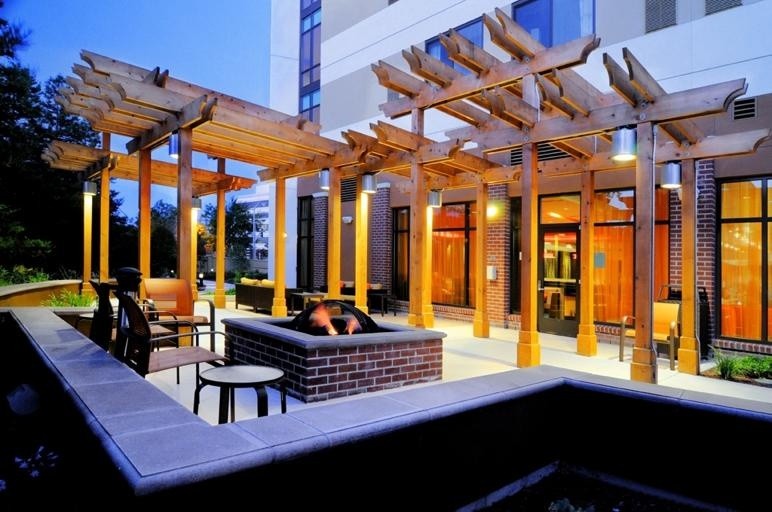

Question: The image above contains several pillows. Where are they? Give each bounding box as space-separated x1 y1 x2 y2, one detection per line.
259 280 276 288
239 276 258 286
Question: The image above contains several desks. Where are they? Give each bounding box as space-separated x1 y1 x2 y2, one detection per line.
368 294 396 317
193 364 288 426
75 313 94 329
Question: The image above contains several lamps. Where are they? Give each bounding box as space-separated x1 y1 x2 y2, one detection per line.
610 129 637 158
660 162 681 187
192 196 201 208
428 192 441 206
83 181 97 194
319 170 329 188
362 175 376 192
169 131 179 155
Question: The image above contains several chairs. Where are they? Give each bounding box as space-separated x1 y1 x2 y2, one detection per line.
88 279 235 423
620 301 681 371
235 285 388 314
